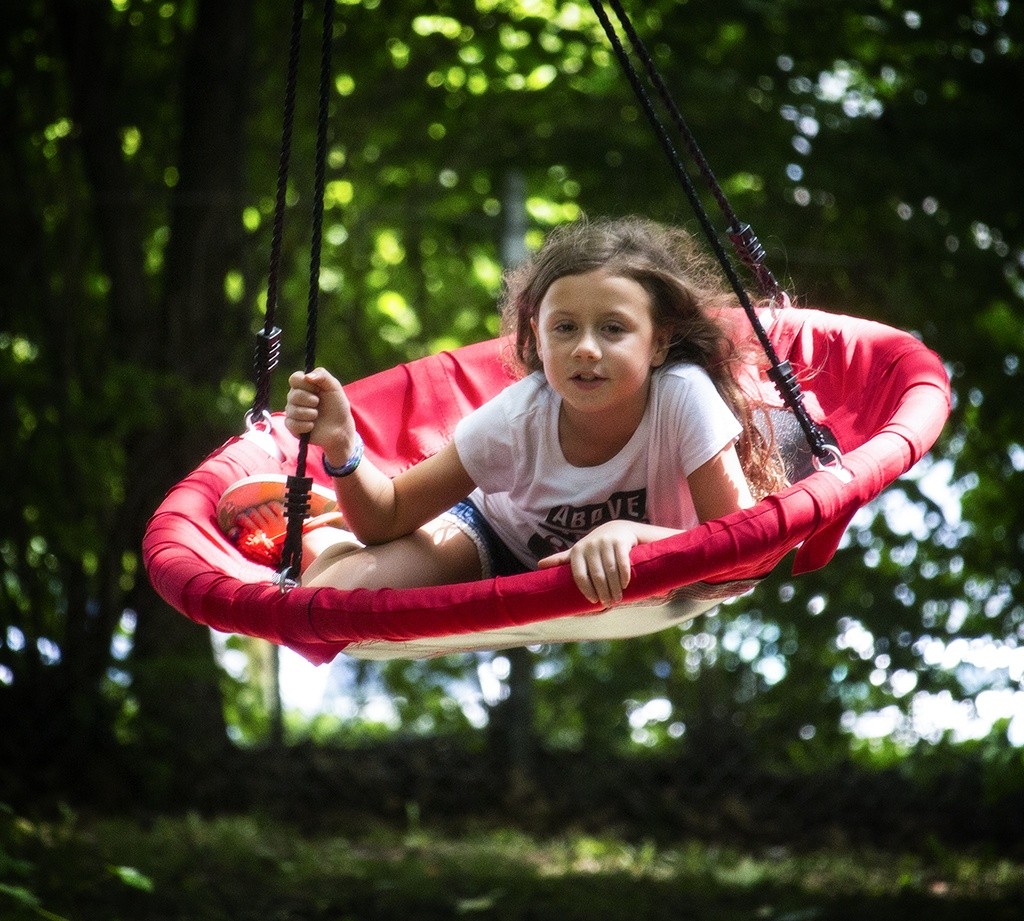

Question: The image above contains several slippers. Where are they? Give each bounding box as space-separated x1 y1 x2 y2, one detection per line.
216 473 350 573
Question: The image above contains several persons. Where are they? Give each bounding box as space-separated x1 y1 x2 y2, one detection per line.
215 219 784 606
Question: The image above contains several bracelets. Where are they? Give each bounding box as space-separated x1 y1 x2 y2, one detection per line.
321 432 364 476
